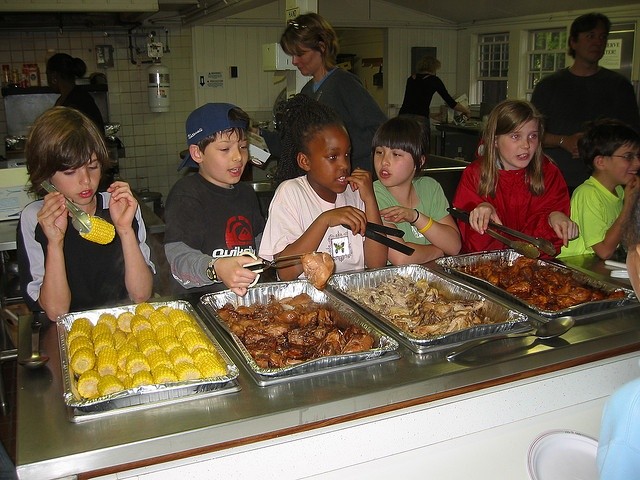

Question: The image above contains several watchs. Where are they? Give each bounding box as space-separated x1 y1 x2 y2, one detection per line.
206 257 225 284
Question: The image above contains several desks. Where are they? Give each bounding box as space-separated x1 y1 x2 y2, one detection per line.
432 116 488 156
250 151 472 193
0 168 165 361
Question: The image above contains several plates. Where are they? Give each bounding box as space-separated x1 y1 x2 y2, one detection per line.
529 431 599 480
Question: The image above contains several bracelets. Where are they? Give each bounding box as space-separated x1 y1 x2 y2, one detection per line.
417 216 433 234
558 135 568 148
409 209 418 223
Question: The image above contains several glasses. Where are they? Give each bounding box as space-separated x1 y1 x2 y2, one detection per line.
287 20 313 31
601 154 640 160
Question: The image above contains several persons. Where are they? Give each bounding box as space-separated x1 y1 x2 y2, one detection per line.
397 54 473 119
245 12 388 184
592 191 640 480
553 115 639 260
162 102 268 297
46 51 107 142
450 98 579 260
371 112 463 266
527 10 639 195
15 106 157 323
257 90 389 284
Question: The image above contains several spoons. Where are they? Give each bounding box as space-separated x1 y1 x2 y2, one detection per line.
446 316 575 360
19 321 50 370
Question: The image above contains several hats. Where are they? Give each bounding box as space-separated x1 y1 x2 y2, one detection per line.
177 102 249 172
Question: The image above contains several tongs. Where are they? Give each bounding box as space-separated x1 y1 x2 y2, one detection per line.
41 181 91 233
238 253 311 288
341 222 415 256
446 208 556 259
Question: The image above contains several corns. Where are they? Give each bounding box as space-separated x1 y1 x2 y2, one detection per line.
79 216 116 245
66 301 227 398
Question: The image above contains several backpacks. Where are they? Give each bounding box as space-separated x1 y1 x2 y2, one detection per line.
373 66 383 86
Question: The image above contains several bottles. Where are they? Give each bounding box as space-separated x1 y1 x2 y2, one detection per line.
19 74 25 89
29 64 40 86
22 64 30 88
10 66 18 88
2 65 10 88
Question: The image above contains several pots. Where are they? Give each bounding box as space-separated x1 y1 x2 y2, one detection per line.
137 189 162 218
